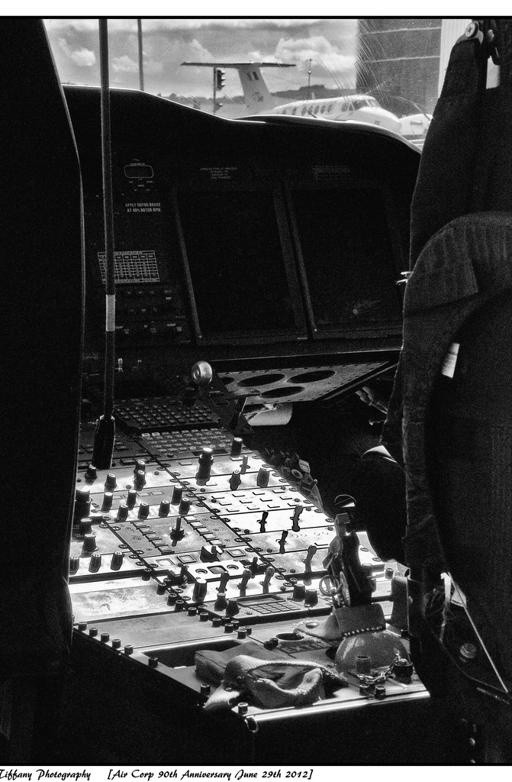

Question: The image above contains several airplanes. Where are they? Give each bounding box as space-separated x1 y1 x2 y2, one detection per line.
177 58 435 148
